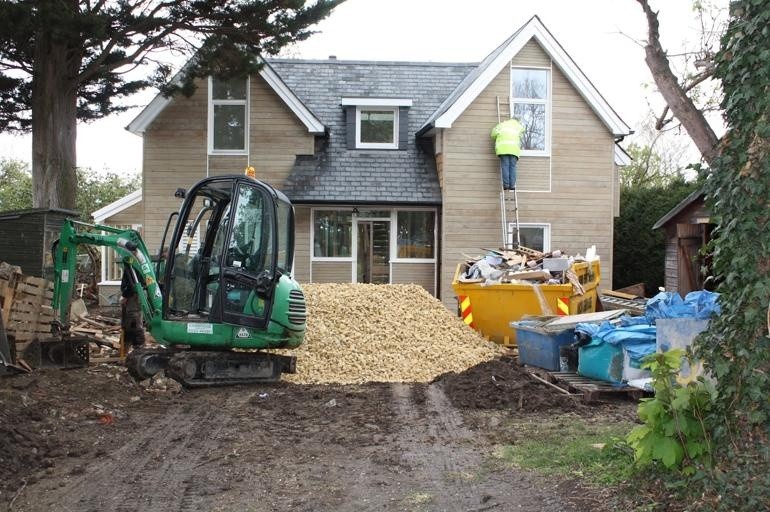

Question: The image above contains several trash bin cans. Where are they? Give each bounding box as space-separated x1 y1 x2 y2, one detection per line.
452 258 600 347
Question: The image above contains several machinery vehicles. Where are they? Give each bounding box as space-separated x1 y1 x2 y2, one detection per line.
20 167 309 390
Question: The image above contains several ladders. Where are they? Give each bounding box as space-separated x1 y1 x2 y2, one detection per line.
497 95 521 250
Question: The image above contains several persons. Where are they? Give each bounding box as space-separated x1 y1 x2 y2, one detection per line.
120 239 147 358
491 114 525 190
156 245 171 293
